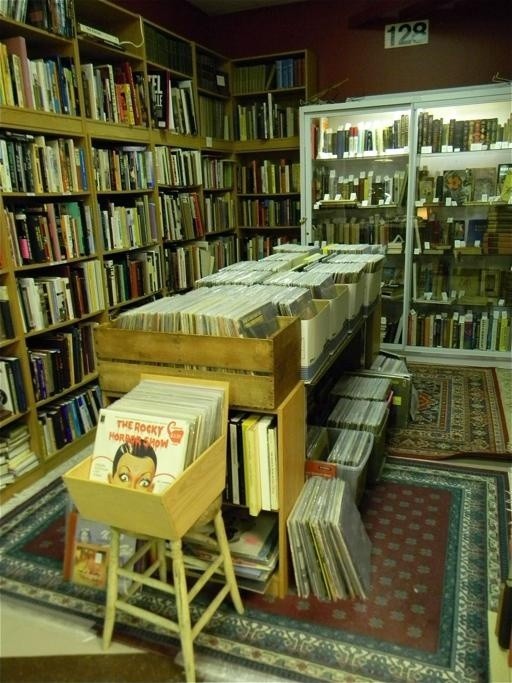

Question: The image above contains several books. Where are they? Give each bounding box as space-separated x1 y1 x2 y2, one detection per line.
0 0 512 602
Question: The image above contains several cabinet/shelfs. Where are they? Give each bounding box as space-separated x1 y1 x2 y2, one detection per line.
0 1 230 506
93 299 380 601
299 82 512 361
230 49 319 262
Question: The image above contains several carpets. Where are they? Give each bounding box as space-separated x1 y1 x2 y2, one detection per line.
384 363 512 460
1 441 512 683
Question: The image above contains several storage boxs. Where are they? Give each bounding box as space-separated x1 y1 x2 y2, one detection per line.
61 435 229 541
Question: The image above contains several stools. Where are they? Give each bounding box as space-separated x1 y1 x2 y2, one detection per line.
103 494 245 682
